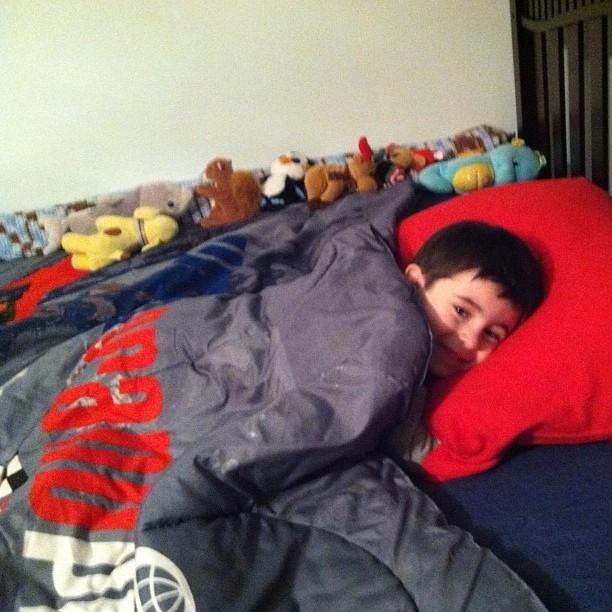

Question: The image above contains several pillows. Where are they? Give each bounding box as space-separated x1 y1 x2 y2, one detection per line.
389 178 611 484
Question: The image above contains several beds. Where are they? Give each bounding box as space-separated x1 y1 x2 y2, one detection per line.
0 123 611 604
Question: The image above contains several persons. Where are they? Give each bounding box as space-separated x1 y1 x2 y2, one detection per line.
403 220 546 382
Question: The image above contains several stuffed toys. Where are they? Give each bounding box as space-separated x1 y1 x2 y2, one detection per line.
62 204 178 271
194 159 260 229
418 137 547 194
258 155 314 212
37 182 193 256
387 146 434 170
425 125 515 161
305 137 377 204
